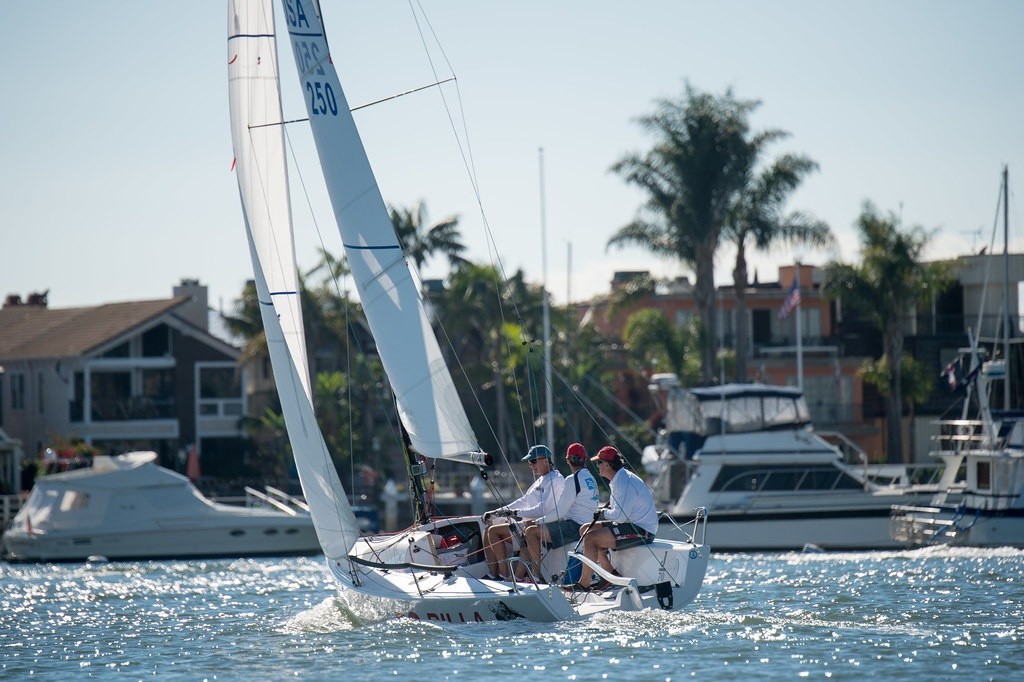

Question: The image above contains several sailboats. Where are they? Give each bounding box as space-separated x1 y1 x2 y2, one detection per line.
891 163 1024 546
227 0 711 624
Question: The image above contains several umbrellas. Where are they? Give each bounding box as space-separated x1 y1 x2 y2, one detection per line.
187 444 199 481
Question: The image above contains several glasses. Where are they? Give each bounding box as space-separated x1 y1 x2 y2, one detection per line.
529 457 546 465
596 460 605 466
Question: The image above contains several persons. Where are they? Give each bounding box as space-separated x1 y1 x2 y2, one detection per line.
500 443 600 584
665 382 705 461
561 445 659 592
479 444 566 582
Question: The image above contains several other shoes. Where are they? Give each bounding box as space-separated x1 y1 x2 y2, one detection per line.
593 569 621 591
565 582 592 592
494 575 506 581
524 575 541 584
481 573 494 580
502 574 524 582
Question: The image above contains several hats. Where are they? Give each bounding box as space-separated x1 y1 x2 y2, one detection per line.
566 442 587 460
521 445 552 462
590 446 620 461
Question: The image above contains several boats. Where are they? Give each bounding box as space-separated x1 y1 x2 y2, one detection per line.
641 373 966 548
2 451 321 563
